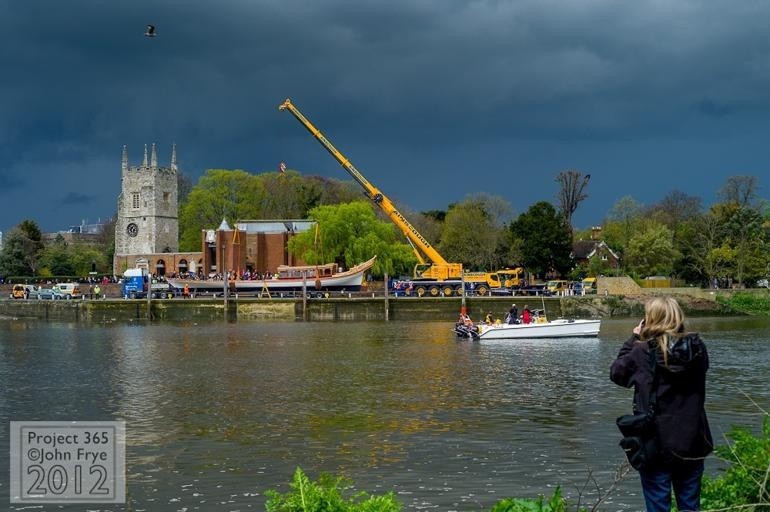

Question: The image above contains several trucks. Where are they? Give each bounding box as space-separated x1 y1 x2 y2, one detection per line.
120 268 170 299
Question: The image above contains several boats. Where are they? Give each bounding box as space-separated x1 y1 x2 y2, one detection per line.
166 254 377 295
452 319 601 339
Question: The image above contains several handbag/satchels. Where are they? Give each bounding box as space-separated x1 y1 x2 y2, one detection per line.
616 412 663 471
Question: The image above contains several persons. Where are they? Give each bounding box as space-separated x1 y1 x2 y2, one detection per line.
509 304 518 324
23 287 30 299
408 281 414 296
88 284 93 299
183 283 189 300
94 284 101 300
486 311 494 325
522 304 536 325
340 287 346 297
34 273 118 285
504 278 511 289
144 282 148 291
144 266 273 284
609 295 714 512
394 280 403 297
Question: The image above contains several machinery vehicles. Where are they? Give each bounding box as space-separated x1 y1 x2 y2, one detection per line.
279 98 463 298
463 268 534 296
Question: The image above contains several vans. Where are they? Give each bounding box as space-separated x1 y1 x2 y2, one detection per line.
543 277 597 295
12 282 83 299
